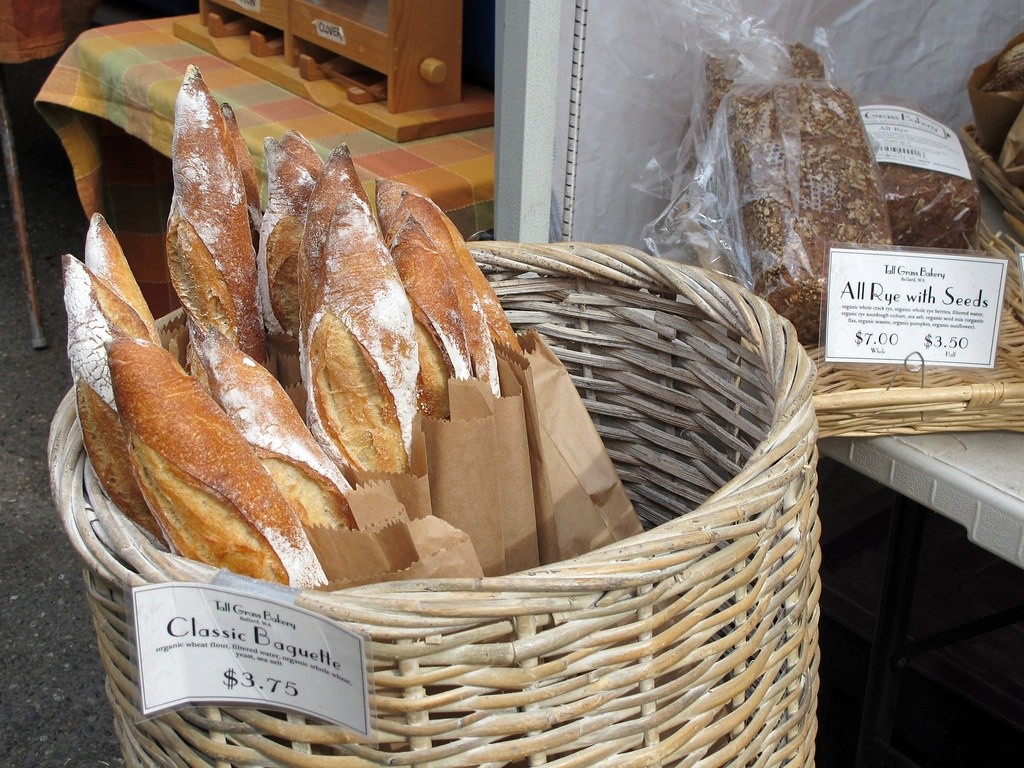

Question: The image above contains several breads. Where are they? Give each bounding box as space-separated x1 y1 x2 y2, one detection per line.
59 63 528 590
707 42 979 345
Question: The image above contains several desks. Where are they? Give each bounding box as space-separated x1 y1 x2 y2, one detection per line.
33 13 493 242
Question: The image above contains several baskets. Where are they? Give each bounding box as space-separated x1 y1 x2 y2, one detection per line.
680 197 1024 440
48 237 819 768
960 122 1024 231
